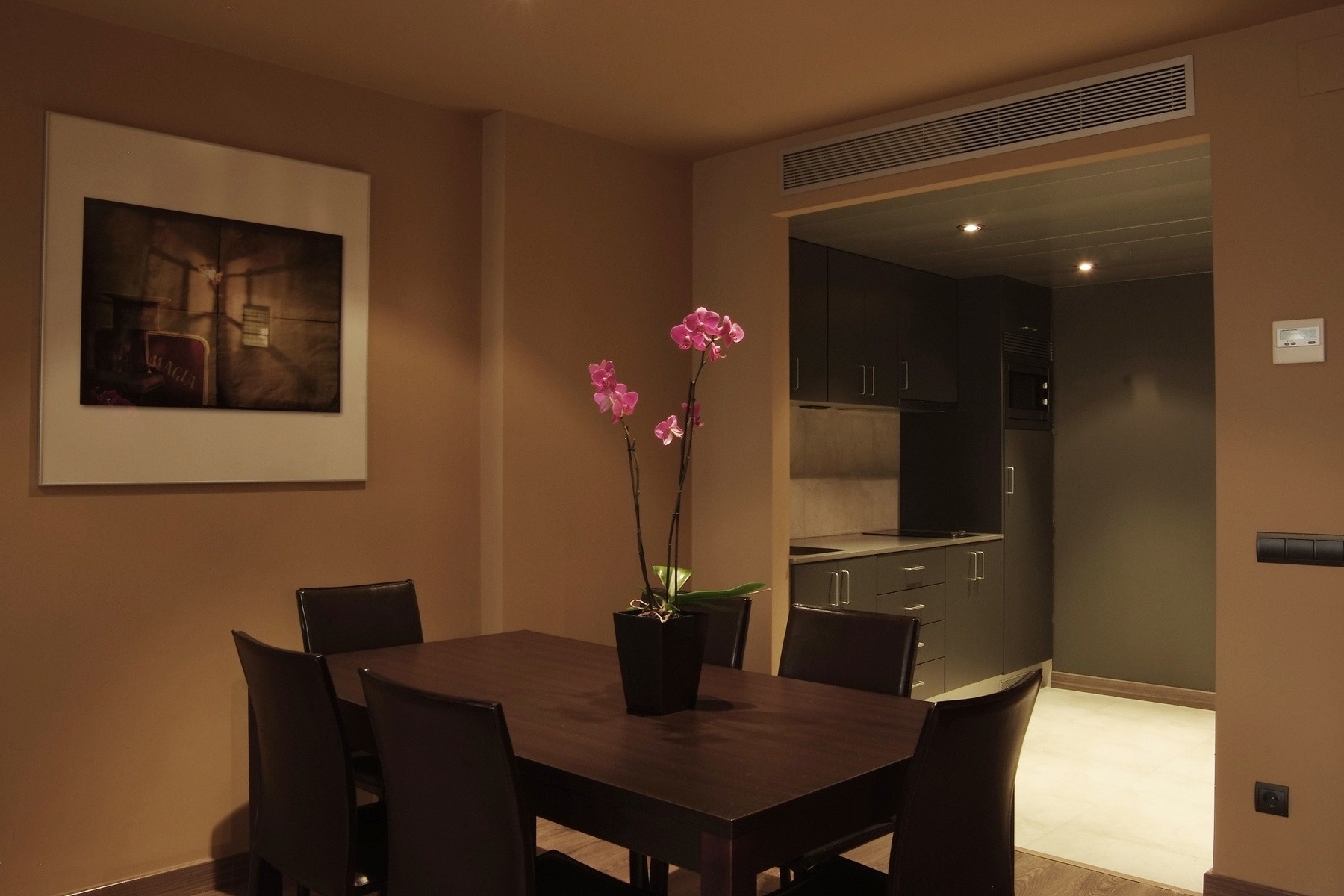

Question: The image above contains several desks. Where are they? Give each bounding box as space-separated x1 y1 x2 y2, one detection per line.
321 630 933 896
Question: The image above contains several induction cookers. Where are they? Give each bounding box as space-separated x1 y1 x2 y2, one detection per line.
861 530 980 539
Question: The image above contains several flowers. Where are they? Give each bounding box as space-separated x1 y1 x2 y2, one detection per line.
589 305 765 625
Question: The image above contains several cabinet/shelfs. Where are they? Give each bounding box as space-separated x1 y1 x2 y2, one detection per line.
789 236 827 408
791 556 877 613
827 247 898 411
876 546 945 700
899 278 1055 673
900 266 958 413
945 539 1005 692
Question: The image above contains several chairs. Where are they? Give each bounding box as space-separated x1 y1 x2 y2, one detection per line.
636 588 752 896
295 578 423 800
358 666 631 896
231 628 388 896
766 667 1042 896
778 602 923 885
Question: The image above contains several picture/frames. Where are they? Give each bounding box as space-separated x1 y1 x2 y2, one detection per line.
38 109 370 485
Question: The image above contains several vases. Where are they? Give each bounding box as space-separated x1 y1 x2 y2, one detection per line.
611 608 707 717
